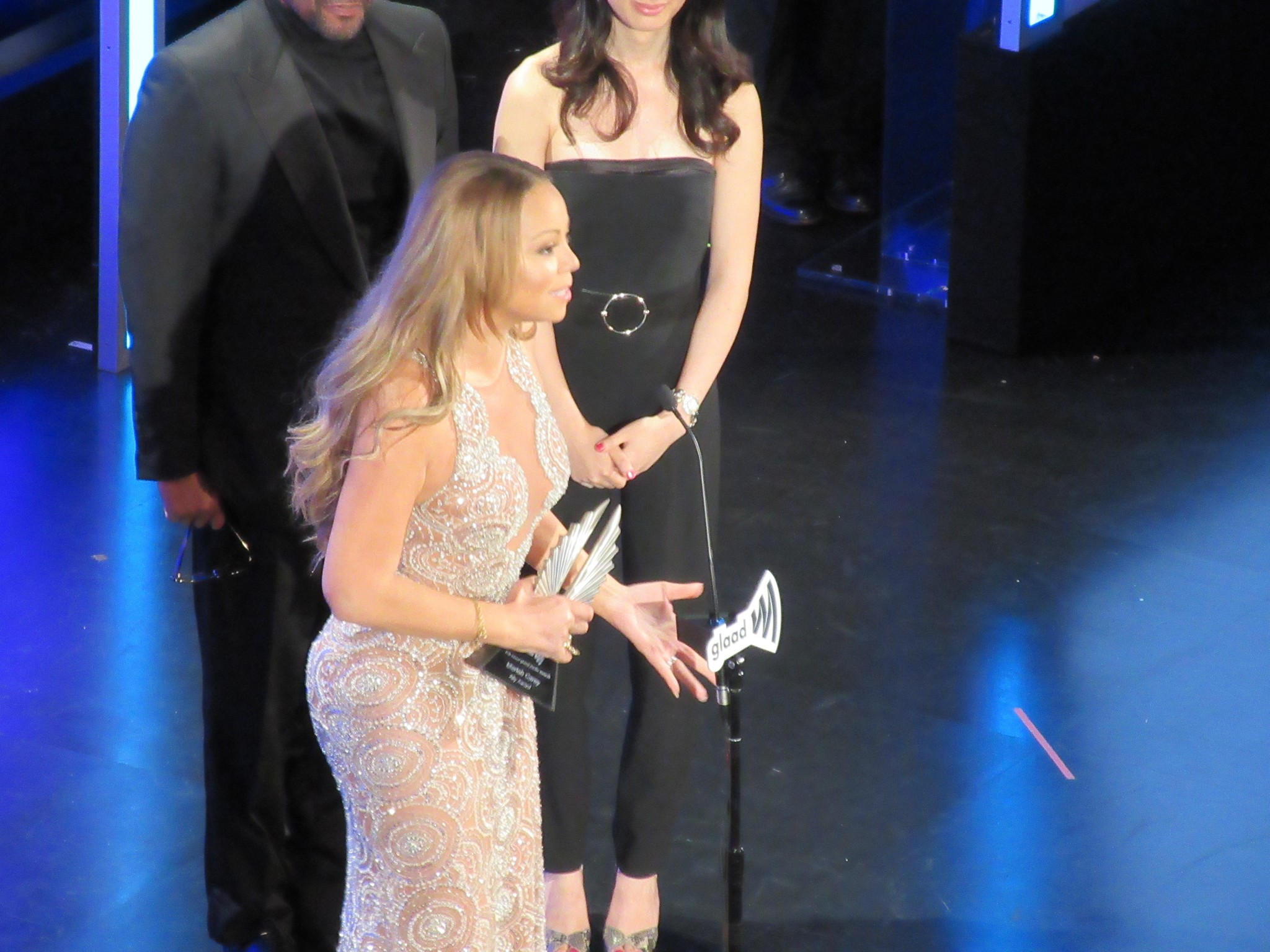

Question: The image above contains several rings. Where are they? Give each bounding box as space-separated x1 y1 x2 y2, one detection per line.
565 634 580 656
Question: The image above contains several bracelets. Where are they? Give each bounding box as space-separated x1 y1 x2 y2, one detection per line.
469 598 487 651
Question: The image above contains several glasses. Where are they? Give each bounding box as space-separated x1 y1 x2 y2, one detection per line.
169 520 253 584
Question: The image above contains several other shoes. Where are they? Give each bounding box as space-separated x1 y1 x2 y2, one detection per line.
546 927 591 952
602 925 658 952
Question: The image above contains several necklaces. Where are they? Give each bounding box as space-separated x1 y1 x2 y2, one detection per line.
452 343 507 388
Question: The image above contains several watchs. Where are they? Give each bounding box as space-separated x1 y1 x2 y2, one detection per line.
672 388 700 429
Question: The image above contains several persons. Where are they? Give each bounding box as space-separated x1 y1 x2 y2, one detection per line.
752 2 881 229
285 152 719 952
494 2 764 952
120 0 459 952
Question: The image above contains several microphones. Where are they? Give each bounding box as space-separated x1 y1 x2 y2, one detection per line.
653 383 730 715
